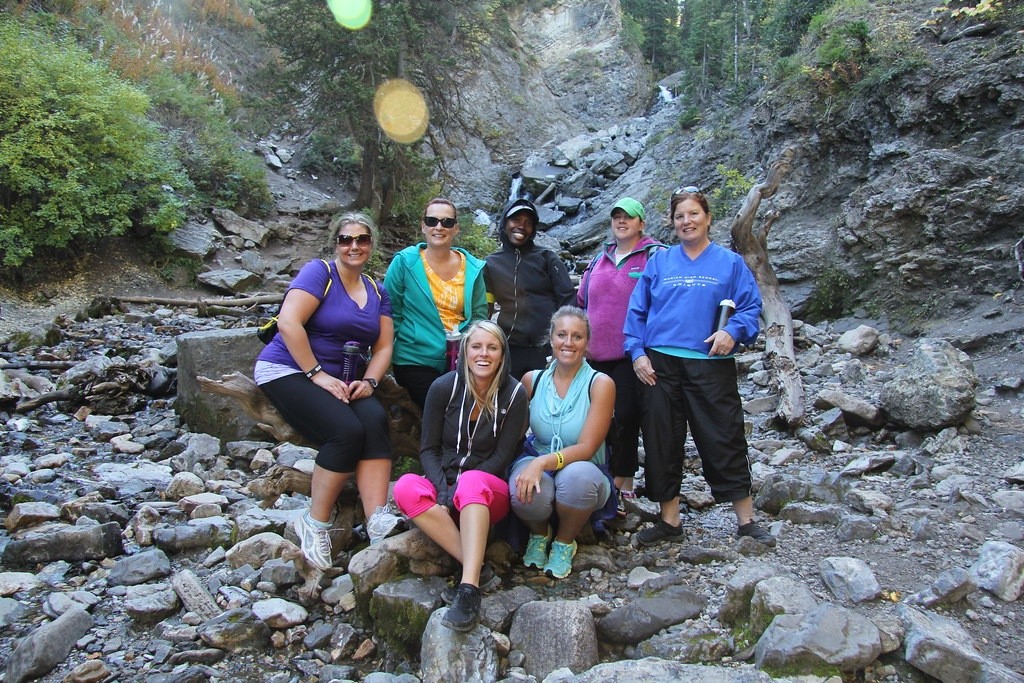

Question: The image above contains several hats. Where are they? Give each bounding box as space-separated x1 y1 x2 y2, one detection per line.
505 200 535 218
609 196 646 221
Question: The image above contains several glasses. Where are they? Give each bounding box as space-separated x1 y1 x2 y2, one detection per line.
334 233 372 247
424 216 457 228
670 185 703 199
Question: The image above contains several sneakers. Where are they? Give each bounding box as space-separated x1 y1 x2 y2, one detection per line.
522 523 553 571
293 506 334 572
734 519 776 547
366 503 409 546
542 536 578 580
636 519 685 547
614 484 626 512
439 583 482 632
440 559 502 605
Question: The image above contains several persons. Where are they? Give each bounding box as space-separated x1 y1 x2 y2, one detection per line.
393 322 529 631
624 190 777 545
255 215 410 571
482 199 577 381
577 198 664 518
509 306 619 579
383 199 489 411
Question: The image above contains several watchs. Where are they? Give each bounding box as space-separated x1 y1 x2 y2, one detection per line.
362 378 379 391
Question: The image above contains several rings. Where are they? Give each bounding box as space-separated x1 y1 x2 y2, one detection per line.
721 351 724 353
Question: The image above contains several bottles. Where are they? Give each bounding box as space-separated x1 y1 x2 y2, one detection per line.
711 299 735 334
444 325 461 373
340 341 360 384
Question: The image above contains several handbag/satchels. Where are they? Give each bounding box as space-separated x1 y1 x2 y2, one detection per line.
256 258 333 346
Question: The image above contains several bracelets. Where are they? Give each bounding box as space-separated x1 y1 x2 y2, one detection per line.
555 452 564 470
306 364 322 378
